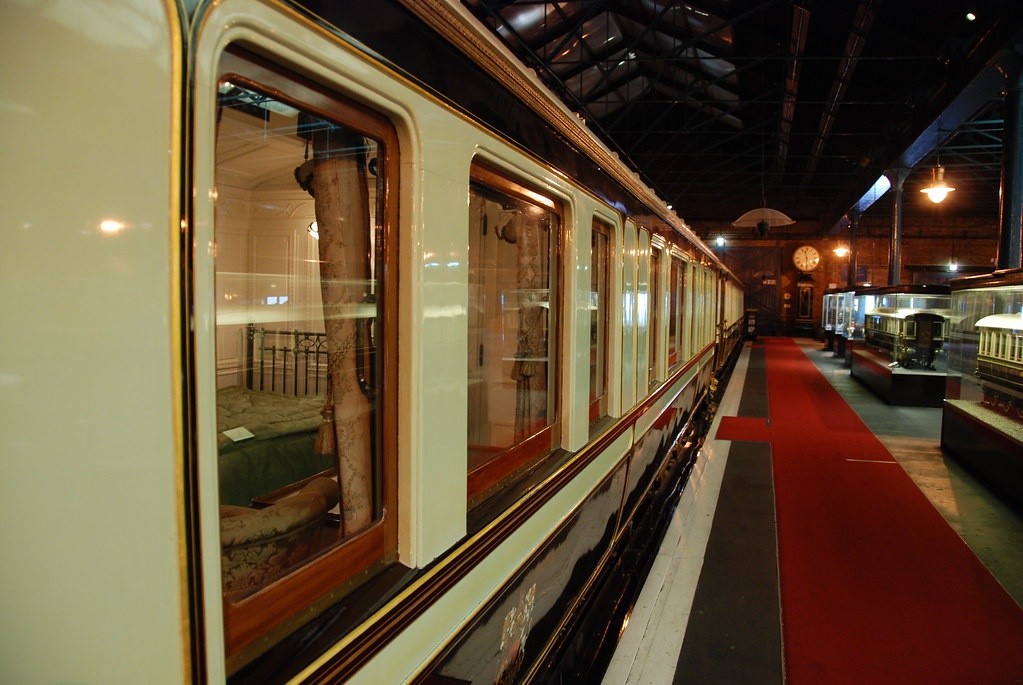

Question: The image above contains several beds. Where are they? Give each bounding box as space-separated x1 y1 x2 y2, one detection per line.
215 322 334 505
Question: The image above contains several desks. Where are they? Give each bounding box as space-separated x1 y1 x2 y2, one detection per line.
252 465 344 528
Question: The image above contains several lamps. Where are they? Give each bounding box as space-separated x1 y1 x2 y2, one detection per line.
918 153 956 204
730 150 797 241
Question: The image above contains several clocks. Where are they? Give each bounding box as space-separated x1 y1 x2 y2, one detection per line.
790 244 823 272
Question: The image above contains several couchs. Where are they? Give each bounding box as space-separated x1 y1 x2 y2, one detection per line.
220 478 340 604
